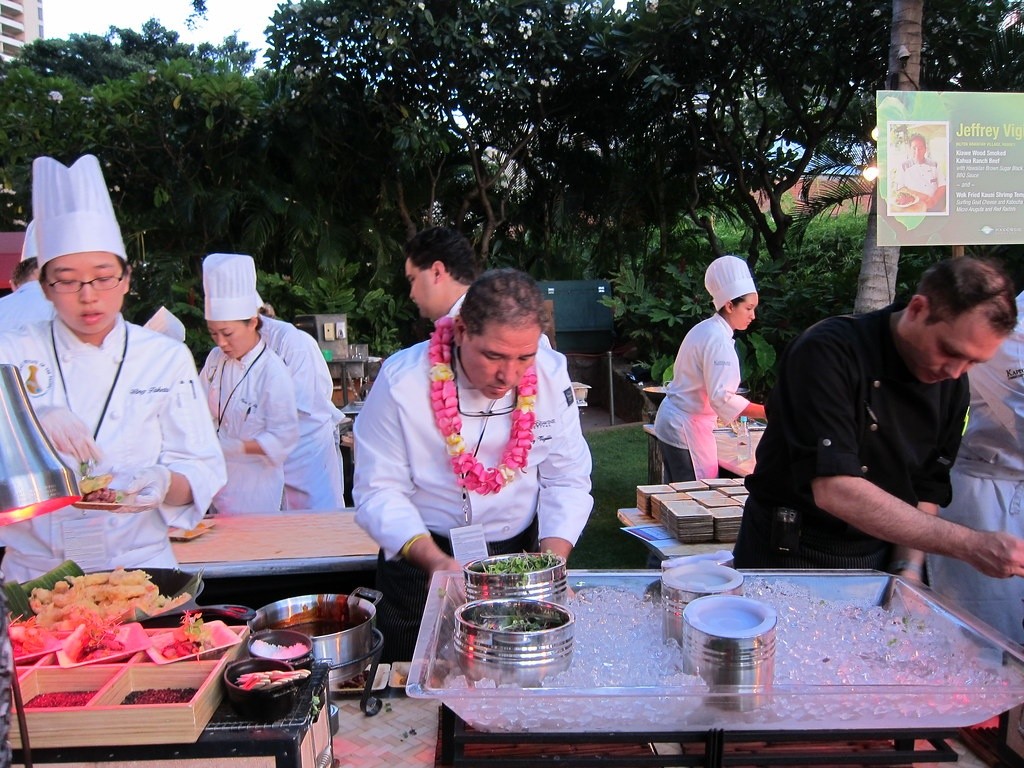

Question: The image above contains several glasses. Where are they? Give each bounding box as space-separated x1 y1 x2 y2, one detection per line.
452 346 518 418
44 272 125 292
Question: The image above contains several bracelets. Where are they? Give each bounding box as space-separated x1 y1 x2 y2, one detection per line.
401 534 424 560
892 561 922 575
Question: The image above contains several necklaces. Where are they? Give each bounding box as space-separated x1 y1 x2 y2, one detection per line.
428 317 538 496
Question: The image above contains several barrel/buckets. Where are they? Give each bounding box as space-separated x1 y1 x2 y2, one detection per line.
657 550 778 714
452 552 571 694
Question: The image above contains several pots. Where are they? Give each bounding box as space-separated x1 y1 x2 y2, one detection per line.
40 568 257 628
643 381 751 414
249 585 384 688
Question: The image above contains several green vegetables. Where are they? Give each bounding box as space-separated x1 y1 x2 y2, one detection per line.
478 548 562 573
497 616 553 632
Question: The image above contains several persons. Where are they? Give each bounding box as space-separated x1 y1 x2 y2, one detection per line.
0 154 228 584
895 133 947 212
654 256 768 485
404 227 480 320
732 256 1024 580
0 219 57 324
257 313 347 511
351 270 594 663
198 253 299 514
926 290 1024 649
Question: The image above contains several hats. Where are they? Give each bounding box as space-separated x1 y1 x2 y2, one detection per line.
19 219 37 263
704 255 757 312
143 306 185 343
202 253 264 320
32 154 129 271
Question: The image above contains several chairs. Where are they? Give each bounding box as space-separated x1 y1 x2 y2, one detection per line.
556 331 617 428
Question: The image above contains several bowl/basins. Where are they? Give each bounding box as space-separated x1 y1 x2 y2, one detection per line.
222 659 295 720
247 629 313 668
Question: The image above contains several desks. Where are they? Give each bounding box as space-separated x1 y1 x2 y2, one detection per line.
163 504 382 613
642 424 767 486
340 401 365 465
616 506 737 567
328 665 991 768
326 357 381 407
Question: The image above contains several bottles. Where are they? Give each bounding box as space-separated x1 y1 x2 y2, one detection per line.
736 416 751 460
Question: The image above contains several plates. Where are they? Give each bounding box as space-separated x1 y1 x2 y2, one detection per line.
330 662 390 697
142 621 243 665
8 627 64 663
388 659 446 688
56 622 150 668
893 194 921 206
70 488 155 511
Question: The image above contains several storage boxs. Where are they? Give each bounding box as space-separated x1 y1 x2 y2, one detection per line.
534 279 616 332
406 567 1024 733
